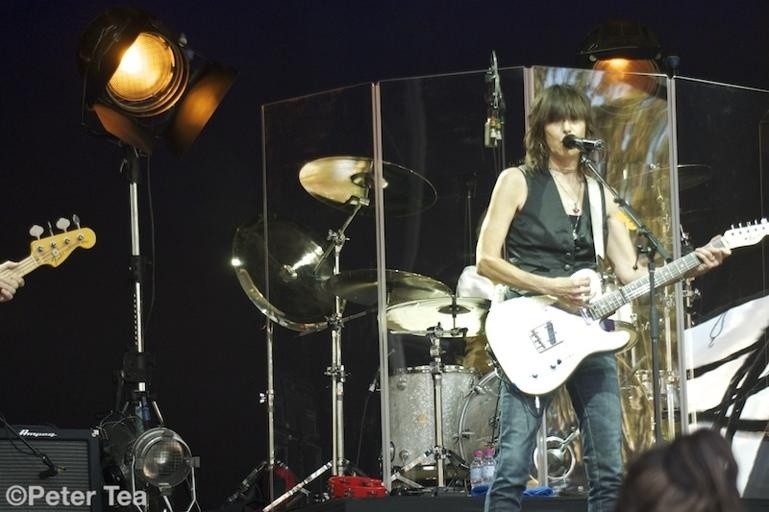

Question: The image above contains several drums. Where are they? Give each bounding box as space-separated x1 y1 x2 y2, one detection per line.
390 363 483 480
458 367 557 477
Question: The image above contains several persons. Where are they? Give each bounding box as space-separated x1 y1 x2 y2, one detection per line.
616 427 744 512
456 209 508 375
476 82 732 512
0 260 25 303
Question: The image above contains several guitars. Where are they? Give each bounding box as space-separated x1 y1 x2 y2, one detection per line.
484 217 768 396
0 213 97 301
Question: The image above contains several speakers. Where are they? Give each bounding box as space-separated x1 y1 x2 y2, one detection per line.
0 421 105 511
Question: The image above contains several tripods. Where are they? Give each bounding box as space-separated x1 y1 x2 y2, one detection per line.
104 183 203 511
392 354 470 491
260 219 388 511
219 335 312 512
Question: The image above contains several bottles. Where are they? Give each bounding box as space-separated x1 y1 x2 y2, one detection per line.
470 449 486 491
481 448 497 484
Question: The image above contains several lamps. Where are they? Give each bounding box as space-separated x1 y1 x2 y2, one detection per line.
68 7 240 511
579 20 665 118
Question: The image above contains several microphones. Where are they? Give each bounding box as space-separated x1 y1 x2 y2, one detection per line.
561 133 605 152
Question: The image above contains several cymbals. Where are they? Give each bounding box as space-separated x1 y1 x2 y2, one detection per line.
230 211 334 333
326 265 452 311
299 156 437 215
387 296 492 339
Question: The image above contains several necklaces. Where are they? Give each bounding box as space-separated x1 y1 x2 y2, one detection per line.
549 167 582 213
